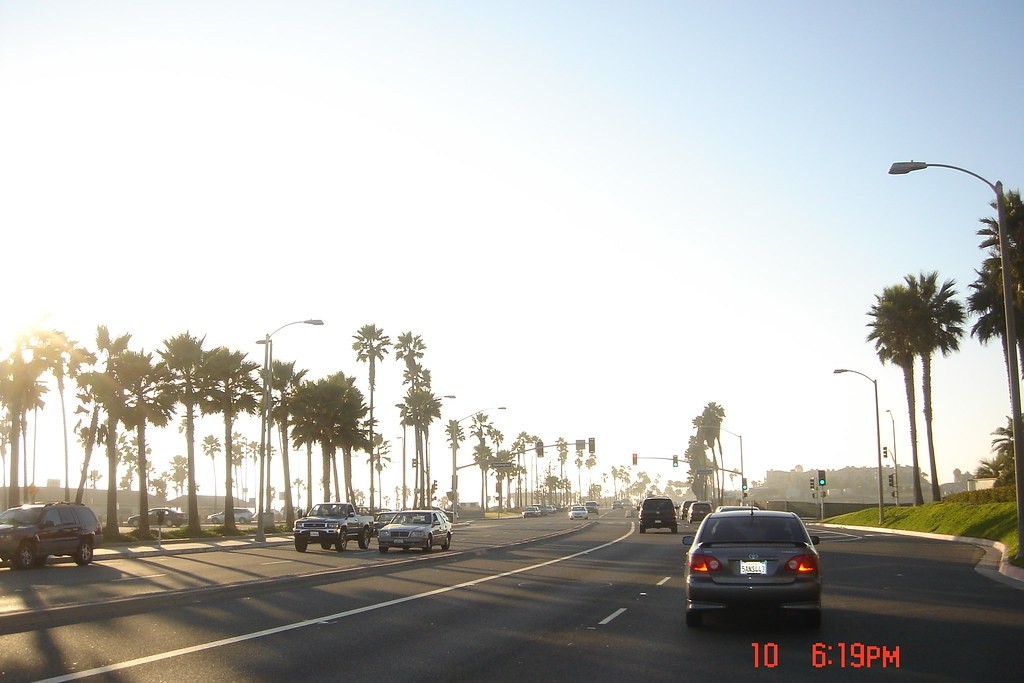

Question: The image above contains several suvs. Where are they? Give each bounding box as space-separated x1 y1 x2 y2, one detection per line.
637 495 713 534
0 501 103 570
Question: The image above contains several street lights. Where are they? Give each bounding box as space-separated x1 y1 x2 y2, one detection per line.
255 318 324 545
420 395 456 511
834 368 884 524
888 160 1024 559
453 407 507 522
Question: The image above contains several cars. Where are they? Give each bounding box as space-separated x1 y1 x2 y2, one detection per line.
522 501 623 520
682 505 822 628
292 502 455 554
127 507 254 528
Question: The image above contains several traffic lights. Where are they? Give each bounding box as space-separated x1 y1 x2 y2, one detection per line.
889 474 893 487
818 470 826 487
742 478 747 490
433 480 438 490
412 459 416 468
883 447 887 458
673 455 678 467
633 454 637 465
810 479 814 489
